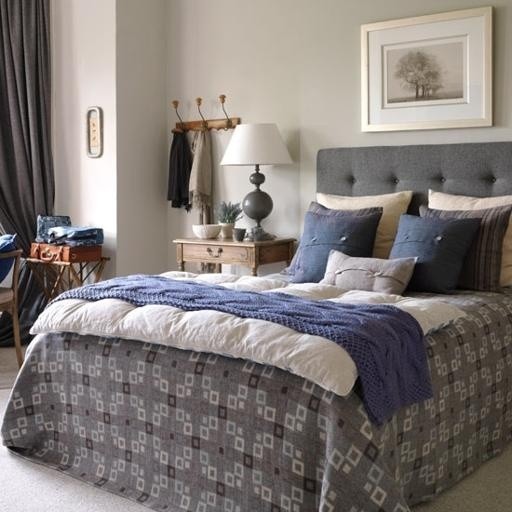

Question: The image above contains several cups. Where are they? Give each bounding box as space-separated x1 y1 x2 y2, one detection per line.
232 228 246 243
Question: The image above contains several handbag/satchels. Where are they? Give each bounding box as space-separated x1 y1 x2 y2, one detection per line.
35 214 105 247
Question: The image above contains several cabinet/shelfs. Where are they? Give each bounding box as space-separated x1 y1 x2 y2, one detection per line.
20 259 107 308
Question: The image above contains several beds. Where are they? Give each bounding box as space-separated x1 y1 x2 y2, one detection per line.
1 141 512 512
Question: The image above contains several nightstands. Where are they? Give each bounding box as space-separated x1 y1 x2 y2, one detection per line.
174 237 297 277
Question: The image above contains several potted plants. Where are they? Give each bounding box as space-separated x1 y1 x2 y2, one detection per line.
215 202 242 240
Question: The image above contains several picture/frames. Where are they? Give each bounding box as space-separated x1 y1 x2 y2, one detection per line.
359 6 492 132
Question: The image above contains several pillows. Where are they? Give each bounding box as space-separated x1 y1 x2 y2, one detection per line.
389 212 483 294
317 249 419 298
419 203 512 294
279 201 383 275
291 211 381 283
427 188 512 290
316 190 413 258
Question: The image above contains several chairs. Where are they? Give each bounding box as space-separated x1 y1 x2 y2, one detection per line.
0 249 23 374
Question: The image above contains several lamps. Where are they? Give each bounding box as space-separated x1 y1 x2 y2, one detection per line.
221 121 296 241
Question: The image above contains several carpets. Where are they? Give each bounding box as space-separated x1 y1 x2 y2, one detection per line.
2 390 155 512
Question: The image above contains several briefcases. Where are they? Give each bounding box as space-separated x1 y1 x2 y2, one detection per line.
30 242 102 263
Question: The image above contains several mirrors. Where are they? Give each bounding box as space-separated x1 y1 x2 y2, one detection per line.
84 106 103 158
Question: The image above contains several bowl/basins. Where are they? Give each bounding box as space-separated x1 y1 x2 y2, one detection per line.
192 224 222 240
